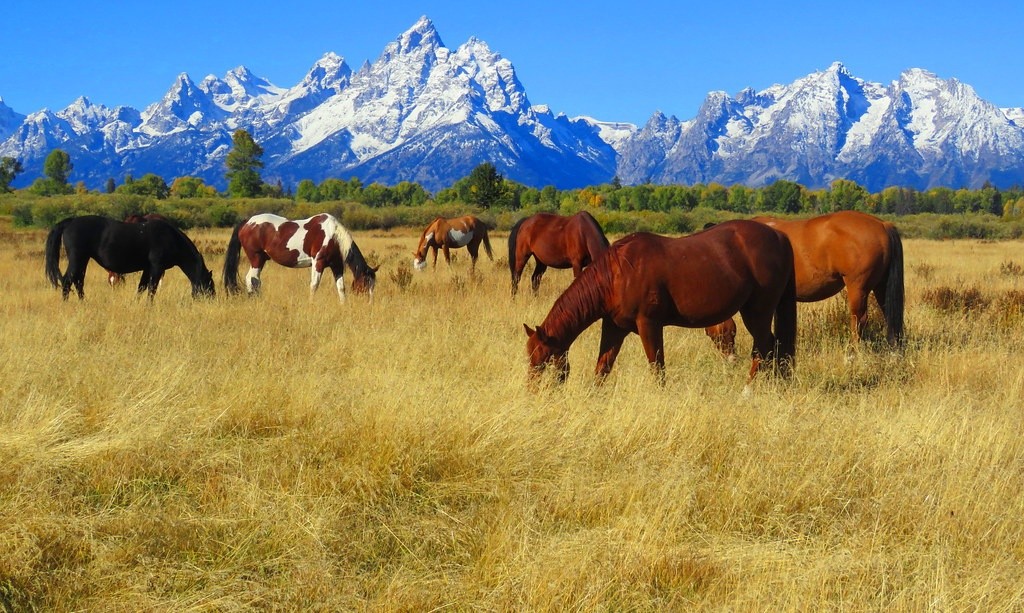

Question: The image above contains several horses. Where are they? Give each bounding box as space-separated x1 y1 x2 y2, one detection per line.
411 215 496 274
507 210 905 396
219 212 382 305
45 212 216 306
